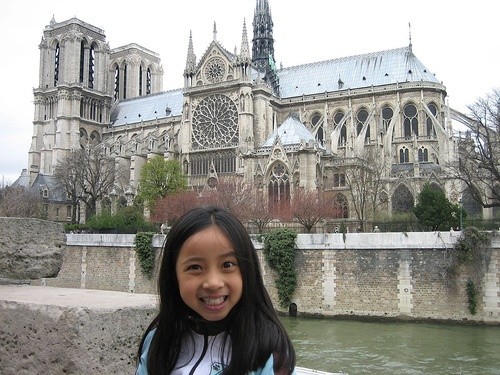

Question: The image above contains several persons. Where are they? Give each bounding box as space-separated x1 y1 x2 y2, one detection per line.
135 206 295 375
